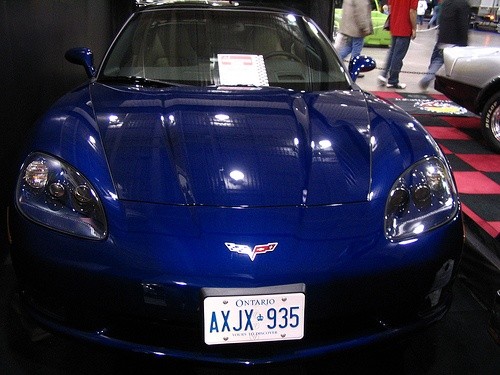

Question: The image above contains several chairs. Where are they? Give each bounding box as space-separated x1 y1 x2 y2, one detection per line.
247 29 287 59
149 26 197 67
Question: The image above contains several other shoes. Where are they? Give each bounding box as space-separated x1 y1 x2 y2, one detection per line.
386 83 406 89
378 75 388 83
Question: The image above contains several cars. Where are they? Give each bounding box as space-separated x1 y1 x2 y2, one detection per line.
0 0 466 375
333 0 392 47
433 45 500 152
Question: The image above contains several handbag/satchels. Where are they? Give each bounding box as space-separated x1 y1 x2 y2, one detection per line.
384 14 390 30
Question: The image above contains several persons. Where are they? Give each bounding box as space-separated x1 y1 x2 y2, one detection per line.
382 4 389 15
427 0 442 29
417 0 473 90
335 0 375 78
416 0 428 29
377 0 418 89
